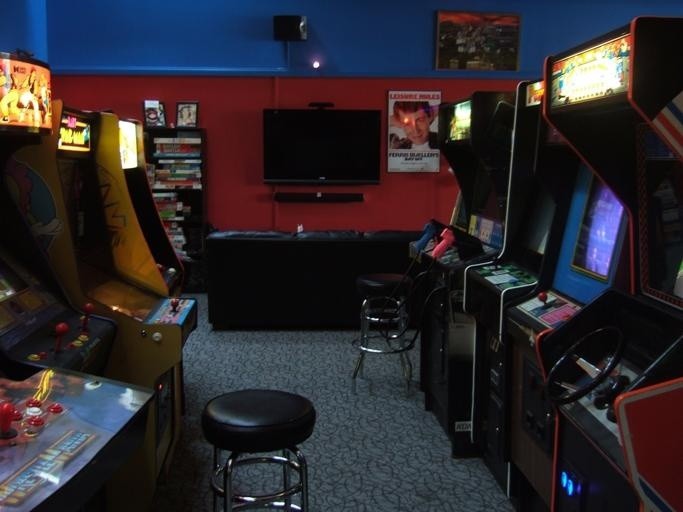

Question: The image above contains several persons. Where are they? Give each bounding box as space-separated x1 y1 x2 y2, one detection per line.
389 100 439 149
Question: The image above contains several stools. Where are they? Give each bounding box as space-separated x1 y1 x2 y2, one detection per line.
351 273 416 380
200 390 316 512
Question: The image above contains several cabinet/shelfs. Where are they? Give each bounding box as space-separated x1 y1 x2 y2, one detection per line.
143 125 207 255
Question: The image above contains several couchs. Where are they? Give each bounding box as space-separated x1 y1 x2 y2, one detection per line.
204 230 424 329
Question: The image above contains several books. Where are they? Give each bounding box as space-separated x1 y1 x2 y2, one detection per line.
143 137 201 263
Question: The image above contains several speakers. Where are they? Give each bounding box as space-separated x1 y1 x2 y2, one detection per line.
273 15 307 41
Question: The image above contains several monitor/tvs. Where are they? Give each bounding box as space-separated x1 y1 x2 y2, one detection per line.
659 238 683 299
522 186 556 265
0 258 32 301
264 108 381 186
571 174 625 284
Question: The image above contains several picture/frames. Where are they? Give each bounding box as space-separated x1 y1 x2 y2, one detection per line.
175 100 199 128
434 9 521 73
142 101 168 128
